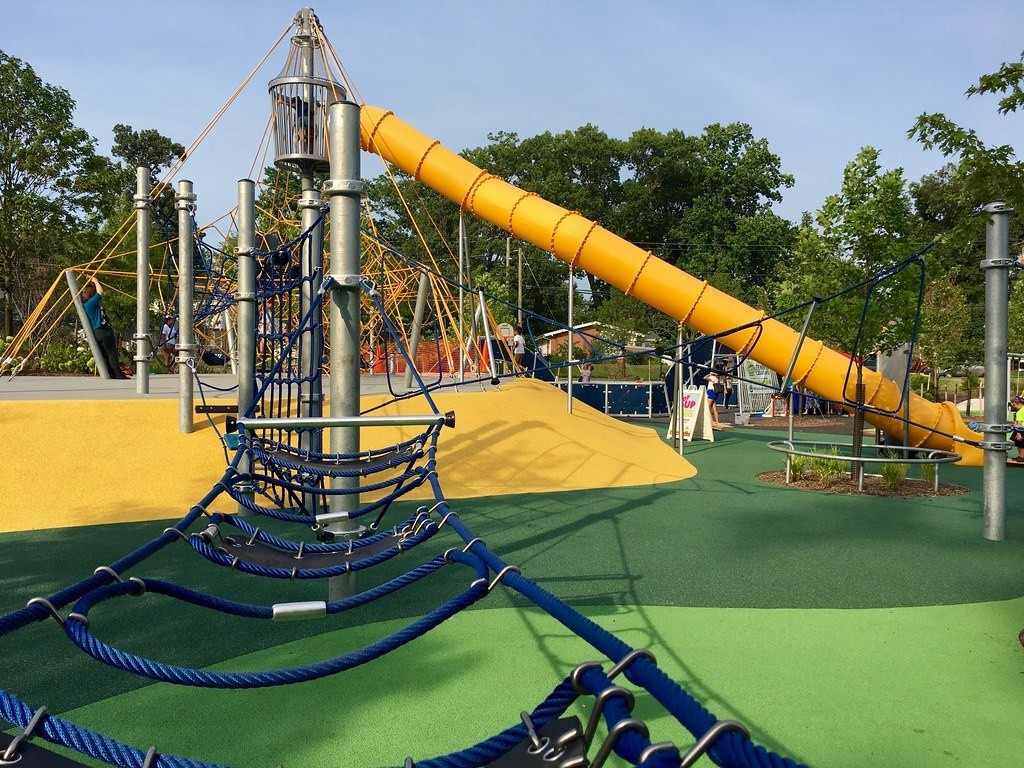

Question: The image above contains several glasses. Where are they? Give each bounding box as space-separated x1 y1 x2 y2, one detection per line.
636 378 639 380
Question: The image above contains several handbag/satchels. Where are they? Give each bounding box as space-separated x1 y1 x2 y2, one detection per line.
712 382 723 393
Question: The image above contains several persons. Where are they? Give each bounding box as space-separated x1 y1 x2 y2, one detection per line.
803 388 820 414
79 275 130 379
781 377 800 393
512 326 528 378
1009 395 1024 462
579 363 594 382
723 374 734 411
703 367 721 424
162 317 179 374
635 375 645 387
276 95 315 154
260 300 296 365
659 373 666 381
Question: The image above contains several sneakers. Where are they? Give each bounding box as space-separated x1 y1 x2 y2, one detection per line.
813 408 817 415
802 409 809 415
1011 455 1024 463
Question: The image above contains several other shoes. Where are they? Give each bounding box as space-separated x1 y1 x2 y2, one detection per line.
724 405 732 410
166 369 175 374
516 367 528 378
112 374 132 380
